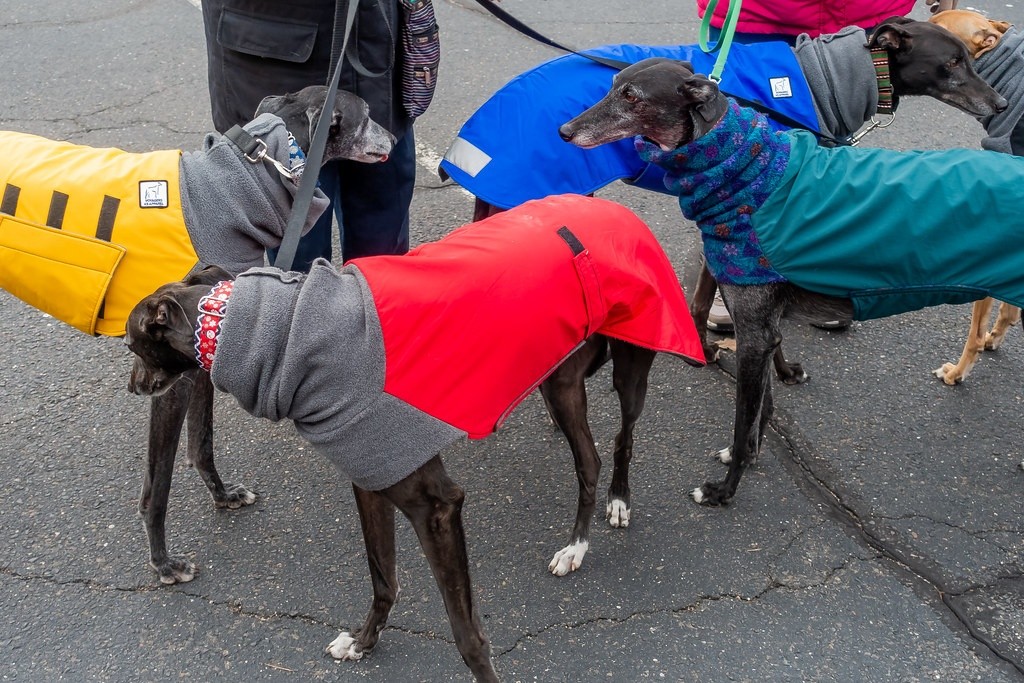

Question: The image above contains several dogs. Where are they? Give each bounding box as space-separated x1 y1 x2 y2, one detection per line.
558 57 1023 506
437 16 1009 367
120 192 709 682
0 85 398 586
926 8 1024 389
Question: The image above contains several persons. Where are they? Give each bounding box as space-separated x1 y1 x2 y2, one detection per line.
201 0 441 276
697 0 959 335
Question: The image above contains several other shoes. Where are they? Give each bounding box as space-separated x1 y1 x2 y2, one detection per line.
708 287 733 324
